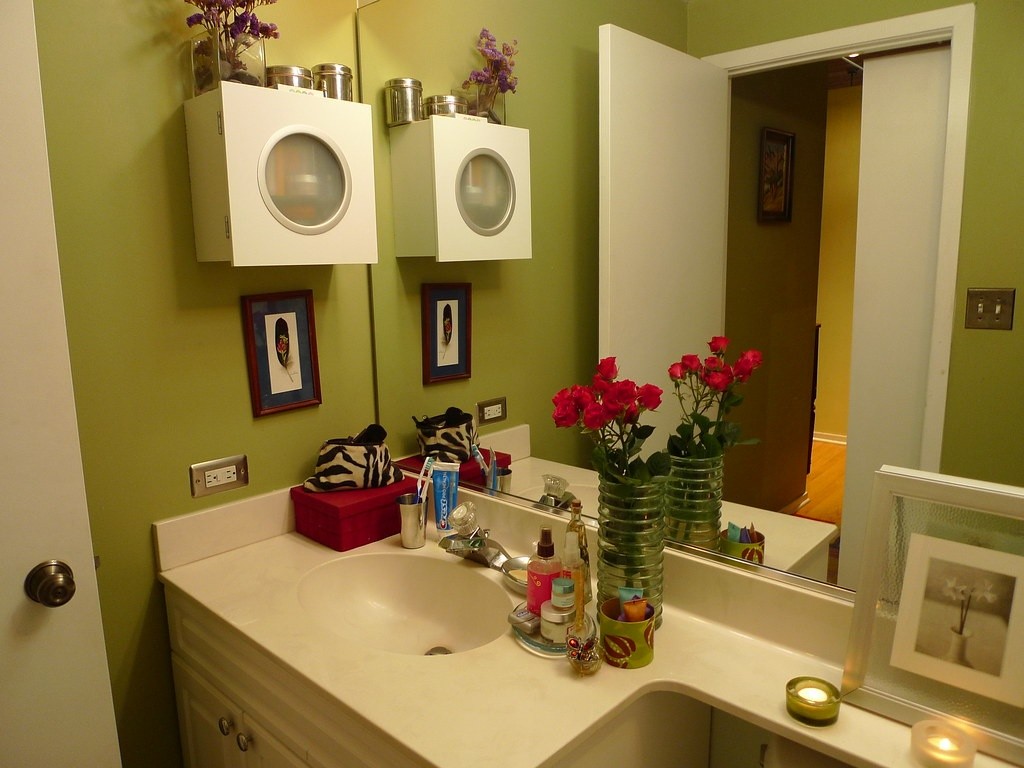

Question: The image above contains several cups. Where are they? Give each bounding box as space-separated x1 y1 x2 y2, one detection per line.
598 599 655 668
395 494 428 548
494 468 513 495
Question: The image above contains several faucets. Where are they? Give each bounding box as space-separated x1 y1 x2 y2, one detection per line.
438 500 510 570
540 474 583 511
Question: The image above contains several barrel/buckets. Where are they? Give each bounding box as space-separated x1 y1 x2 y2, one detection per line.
384 77 469 127
267 63 353 101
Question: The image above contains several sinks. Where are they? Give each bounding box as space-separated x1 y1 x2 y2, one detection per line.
297 552 516 656
520 483 601 517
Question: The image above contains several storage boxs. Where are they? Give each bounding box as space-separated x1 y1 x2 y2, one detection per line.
291 474 426 553
392 447 512 492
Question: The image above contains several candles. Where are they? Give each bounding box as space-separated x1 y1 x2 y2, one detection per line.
785 676 841 728
911 719 978 768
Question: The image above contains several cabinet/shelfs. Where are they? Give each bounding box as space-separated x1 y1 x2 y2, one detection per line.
182 80 378 267
387 114 532 263
163 585 711 768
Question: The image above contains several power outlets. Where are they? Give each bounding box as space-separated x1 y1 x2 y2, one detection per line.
475 396 508 426
189 454 249 499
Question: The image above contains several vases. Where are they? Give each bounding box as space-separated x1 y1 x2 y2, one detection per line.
452 83 507 125
661 447 726 553
596 474 668 631
190 27 267 98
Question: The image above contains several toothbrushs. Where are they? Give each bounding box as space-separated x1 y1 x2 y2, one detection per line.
414 456 439 503
470 443 489 476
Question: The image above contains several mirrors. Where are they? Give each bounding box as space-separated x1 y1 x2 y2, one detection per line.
352 0 1024 736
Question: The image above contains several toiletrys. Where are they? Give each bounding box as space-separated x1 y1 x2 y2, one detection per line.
486 447 500 492
523 523 562 615
618 585 647 623
430 461 460 546
560 530 584 632
724 521 757 544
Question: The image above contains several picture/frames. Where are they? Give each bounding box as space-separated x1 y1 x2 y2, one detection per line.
756 125 796 222
419 282 472 386
838 469 1024 768
240 288 323 418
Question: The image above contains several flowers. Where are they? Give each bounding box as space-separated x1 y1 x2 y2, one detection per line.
551 356 671 600
461 28 519 117
666 334 763 541
183 0 281 93
